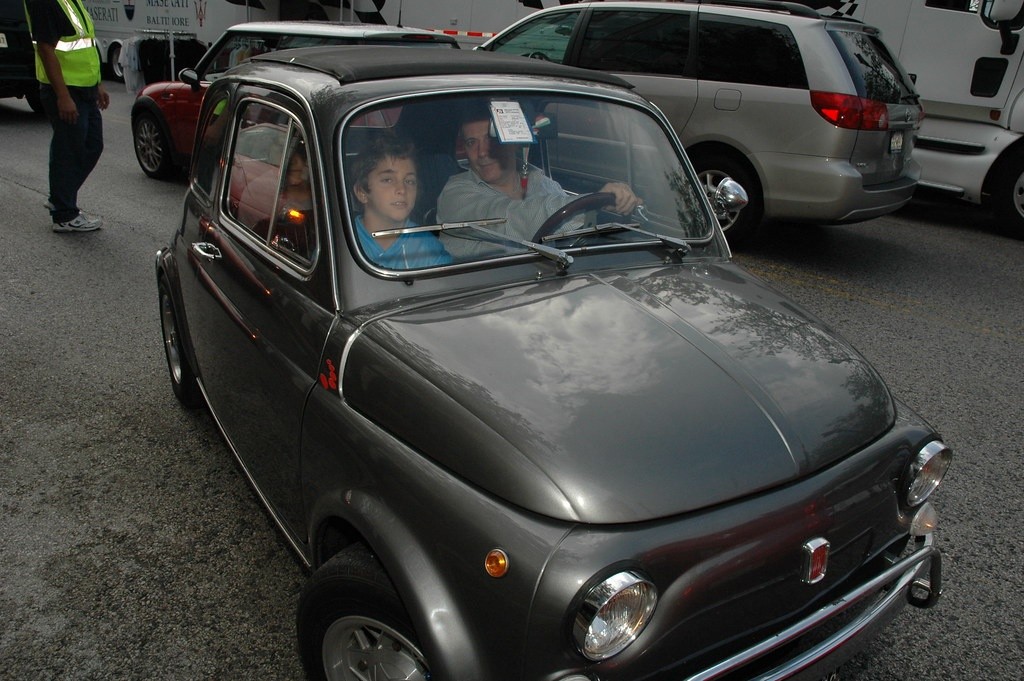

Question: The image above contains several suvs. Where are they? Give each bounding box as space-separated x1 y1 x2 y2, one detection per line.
471 3 925 249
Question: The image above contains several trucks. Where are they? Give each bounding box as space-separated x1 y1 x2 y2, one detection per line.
78 0 582 84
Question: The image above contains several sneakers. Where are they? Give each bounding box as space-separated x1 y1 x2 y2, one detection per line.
42 198 57 211
52 212 103 231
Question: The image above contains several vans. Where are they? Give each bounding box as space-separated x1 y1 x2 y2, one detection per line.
811 0 1024 240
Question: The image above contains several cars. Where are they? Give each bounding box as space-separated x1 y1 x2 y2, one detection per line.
130 21 462 183
0 0 54 123
152 44 955 681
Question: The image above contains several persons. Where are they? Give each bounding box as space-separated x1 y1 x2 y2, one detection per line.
354 113 644 272
24 1 110 232
